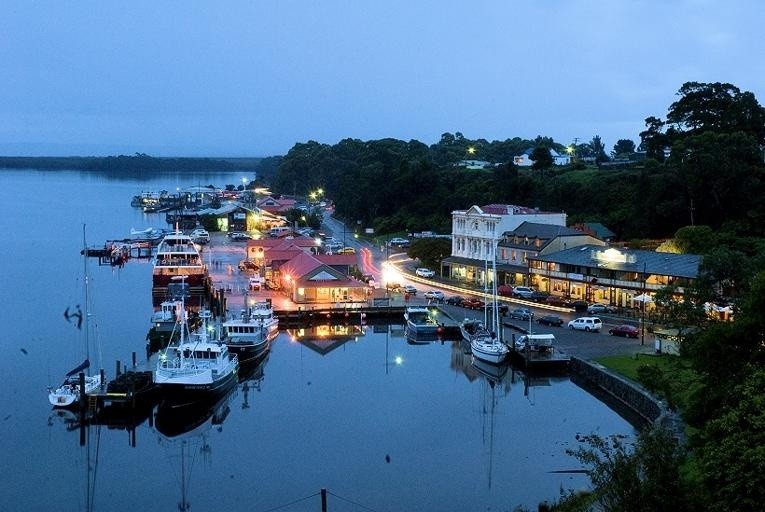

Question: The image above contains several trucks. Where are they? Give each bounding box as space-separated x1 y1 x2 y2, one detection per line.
515 334 554 351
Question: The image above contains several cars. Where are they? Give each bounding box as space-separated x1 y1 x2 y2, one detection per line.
268 226 356 255
609 325 639 338
395 283 617 333
389 237 410 244
415 268 434 279
295 198 335 215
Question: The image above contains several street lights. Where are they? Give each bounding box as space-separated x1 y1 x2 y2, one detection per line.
340 224 358 255
384 331 402 377
243 177 246 191
464 147 475 166
317 188 323 209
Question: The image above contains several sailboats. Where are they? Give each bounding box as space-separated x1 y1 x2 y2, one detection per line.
469 358 512 491
468 238 508 366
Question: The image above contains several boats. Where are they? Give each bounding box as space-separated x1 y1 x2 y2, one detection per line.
402 305 439 343
152 380 241 512
457 316 486 343
403 326 438 345
